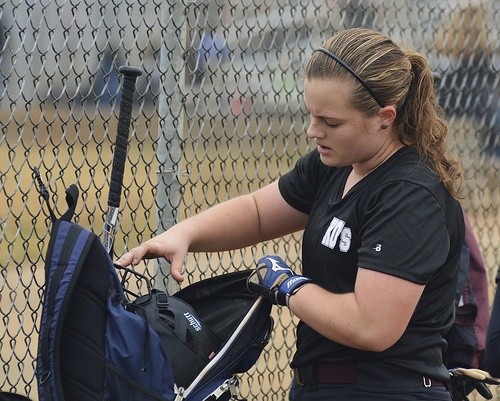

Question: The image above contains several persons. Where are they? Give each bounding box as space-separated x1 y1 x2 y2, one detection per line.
116 27 465 401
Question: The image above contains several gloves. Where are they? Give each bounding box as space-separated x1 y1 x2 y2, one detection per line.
247 254 312 306
449 368 500 401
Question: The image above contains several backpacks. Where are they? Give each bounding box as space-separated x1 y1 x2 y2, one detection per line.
33 167 274 401
